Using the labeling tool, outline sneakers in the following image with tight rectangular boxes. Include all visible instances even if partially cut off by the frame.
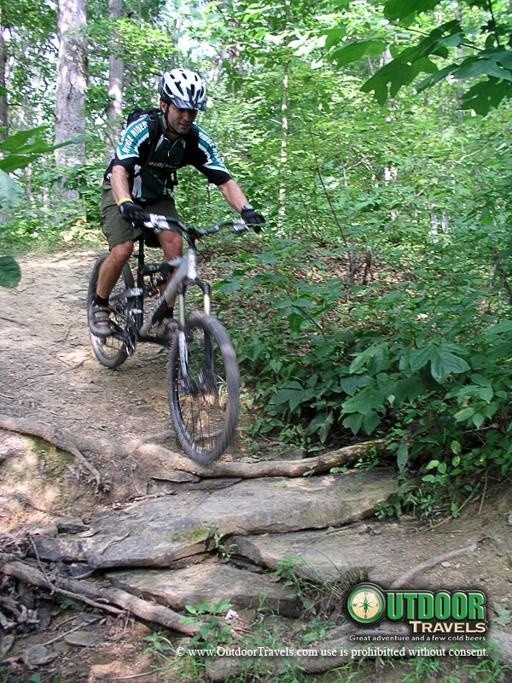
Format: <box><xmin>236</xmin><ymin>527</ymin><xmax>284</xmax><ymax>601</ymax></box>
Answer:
<box><xmin>155</xmin><ymin>317</ymin><xmax>178</xmax><ymax>340</ymax></box>
<box><xmin>88</xmin><ymin>302</ymin><xmax>114</xmax><ymax>339</ymax></box>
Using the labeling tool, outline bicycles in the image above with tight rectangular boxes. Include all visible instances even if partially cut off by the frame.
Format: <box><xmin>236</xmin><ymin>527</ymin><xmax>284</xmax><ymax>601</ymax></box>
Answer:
<box><xmin>87</xmin><ymin>205</ymin><xmax>264</xmax><ymax>466</ymax></box>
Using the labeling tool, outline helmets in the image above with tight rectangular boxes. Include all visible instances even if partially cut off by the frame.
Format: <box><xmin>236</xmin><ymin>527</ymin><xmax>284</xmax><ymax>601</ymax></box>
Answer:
<box><xmin>159</xmin><ymin>69</ymin><xmax>207</xmax><ymax>112</ymax></box>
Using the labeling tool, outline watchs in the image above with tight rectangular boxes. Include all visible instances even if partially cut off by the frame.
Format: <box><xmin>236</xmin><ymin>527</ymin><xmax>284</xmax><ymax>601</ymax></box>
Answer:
<box><xmin>241</xmin><ymin>203</ymin><xmax>255</xmax><ymax>215</ymax></box>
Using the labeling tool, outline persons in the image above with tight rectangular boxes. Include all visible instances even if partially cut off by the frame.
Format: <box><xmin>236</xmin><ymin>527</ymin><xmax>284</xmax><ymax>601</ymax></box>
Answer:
<box><xmin>88</xmin><ymin>68</ymin><xmax>265</xmax><ymax>343</ymax></box>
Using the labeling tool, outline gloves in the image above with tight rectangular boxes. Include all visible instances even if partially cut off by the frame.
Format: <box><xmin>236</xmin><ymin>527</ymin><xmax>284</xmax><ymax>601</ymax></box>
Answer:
<box><xmin>117</xmin><ymin>201</ymin><xmax>145</xmax><ymax>222</ymax></box>
<box><xmin>241</xmin><ymin>205</ymin><xmax>266</xmax><ymax>233</ymax></box>
